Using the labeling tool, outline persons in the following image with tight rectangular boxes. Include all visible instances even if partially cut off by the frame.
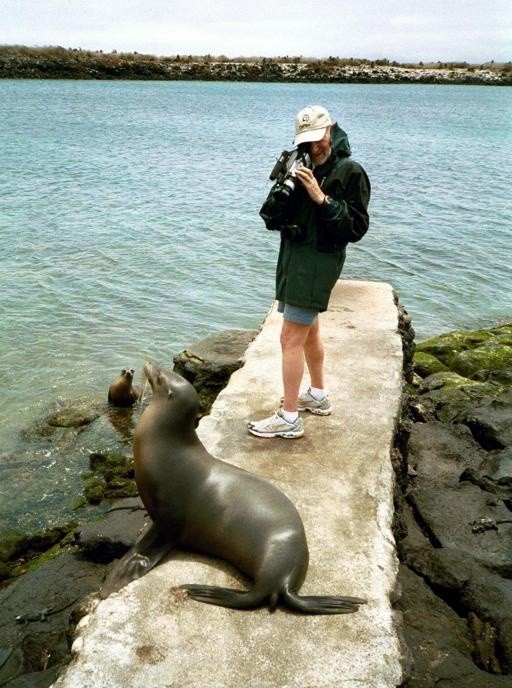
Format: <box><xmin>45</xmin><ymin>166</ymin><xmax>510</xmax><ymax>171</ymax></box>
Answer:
<box><xmin>243</xmin><ymin>104</ymin><xmax>371</xmax><ymax>439</ymax></box>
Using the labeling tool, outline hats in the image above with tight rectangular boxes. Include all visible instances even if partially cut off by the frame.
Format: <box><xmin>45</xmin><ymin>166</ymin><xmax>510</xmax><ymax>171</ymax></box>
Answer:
<box><xmin>293</xmin><ymin>105</ymin><xmax>333</xmax><ymax>147</ymax></box>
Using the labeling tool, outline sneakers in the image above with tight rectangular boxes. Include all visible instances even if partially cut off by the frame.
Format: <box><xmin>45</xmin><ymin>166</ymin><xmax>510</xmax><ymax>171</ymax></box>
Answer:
<box><xmin>280</xmin><ymin>388</ymin><xmax>332</xmax><ymax>416</ymax></box>
<box><xmin>247</xmin><ymin>409</ymin><xmax>305</xmax><ymax>439</ymax></box>
